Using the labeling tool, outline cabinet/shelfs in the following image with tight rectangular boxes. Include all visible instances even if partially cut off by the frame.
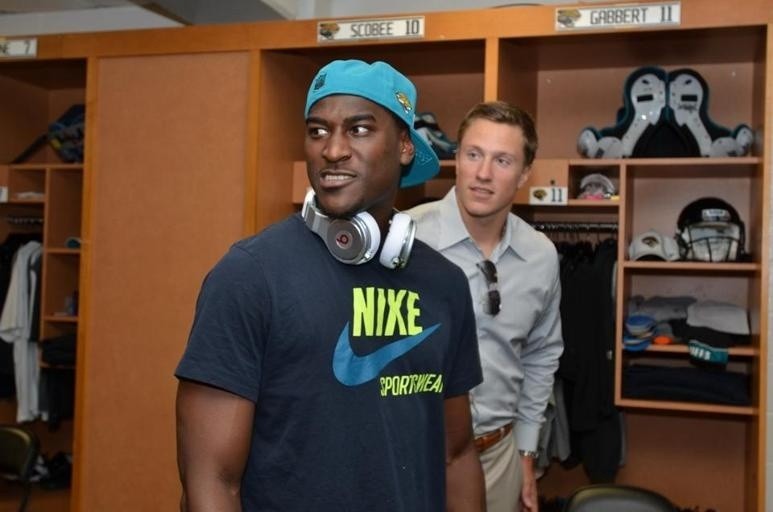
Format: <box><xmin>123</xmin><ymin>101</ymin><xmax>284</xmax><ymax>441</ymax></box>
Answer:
<box><xmin>0</xmin><ymin>0</ymin><xmax>772</xmax><ymax>512</ymax></box>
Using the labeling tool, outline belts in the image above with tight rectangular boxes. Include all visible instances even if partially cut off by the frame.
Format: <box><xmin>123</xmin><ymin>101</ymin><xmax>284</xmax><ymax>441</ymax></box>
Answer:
<box><xmin>474</xmin><ymin>423</ymin><xmax>515</xmax><ymax>452</ymax></box>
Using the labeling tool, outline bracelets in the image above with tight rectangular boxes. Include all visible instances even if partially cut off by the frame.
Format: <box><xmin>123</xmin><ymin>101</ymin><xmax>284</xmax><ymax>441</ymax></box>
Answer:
<box><xmin>519</xmin><ymin>450</ymin><xmax>539</xmax><ymax>459</ymax></box>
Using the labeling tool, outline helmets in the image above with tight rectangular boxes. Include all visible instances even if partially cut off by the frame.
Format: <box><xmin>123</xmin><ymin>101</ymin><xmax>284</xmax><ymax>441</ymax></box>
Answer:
<box><xmin>674</xmin><ymin>197</ymin><xmax>748</xmax><ymax>263</ymax></box>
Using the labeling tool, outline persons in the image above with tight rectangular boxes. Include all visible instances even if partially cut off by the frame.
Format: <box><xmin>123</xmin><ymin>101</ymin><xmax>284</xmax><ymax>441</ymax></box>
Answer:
<box><xmin>172</xmin><ymin>57</ymin><xmax>488</xmax><ymax>512</ymax></box>
<box><xmin>393</xmin><ymin>100</ymin><xmax>564</xmax><ymax>512</ymax></box>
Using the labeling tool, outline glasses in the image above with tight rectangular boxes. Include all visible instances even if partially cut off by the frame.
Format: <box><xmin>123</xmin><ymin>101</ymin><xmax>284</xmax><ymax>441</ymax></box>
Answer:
<box><xmin>476</xmin><ymin>259</ymin><xmax>503</xmax><ymax>317</ymax></box>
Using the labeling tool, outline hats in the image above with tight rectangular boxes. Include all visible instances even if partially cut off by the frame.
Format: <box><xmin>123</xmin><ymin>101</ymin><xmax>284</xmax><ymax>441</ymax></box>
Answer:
<box><xmin>627</xmin><ymin>230</ymin><xmax>674</xmax><ymax>262</ymax></box>
<box><xmin>303</xmin><ymin>58</ymin><xmax>441</xmax><ymax>192</ymax></box>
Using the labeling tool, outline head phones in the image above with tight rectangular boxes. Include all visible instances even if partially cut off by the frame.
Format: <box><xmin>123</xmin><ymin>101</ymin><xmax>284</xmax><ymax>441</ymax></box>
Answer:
<box><xmin>301</xmin><ymin>189</ymin><xmax>418</xmax><ymax>272</ymax></box>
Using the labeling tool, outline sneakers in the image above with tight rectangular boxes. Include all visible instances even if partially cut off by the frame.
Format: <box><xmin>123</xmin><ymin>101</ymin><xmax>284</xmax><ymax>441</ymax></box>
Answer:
<box><xmin>4</xmin><ymin>452</ymin><xmax>73</xmax><ymax>488</ymax></box>
